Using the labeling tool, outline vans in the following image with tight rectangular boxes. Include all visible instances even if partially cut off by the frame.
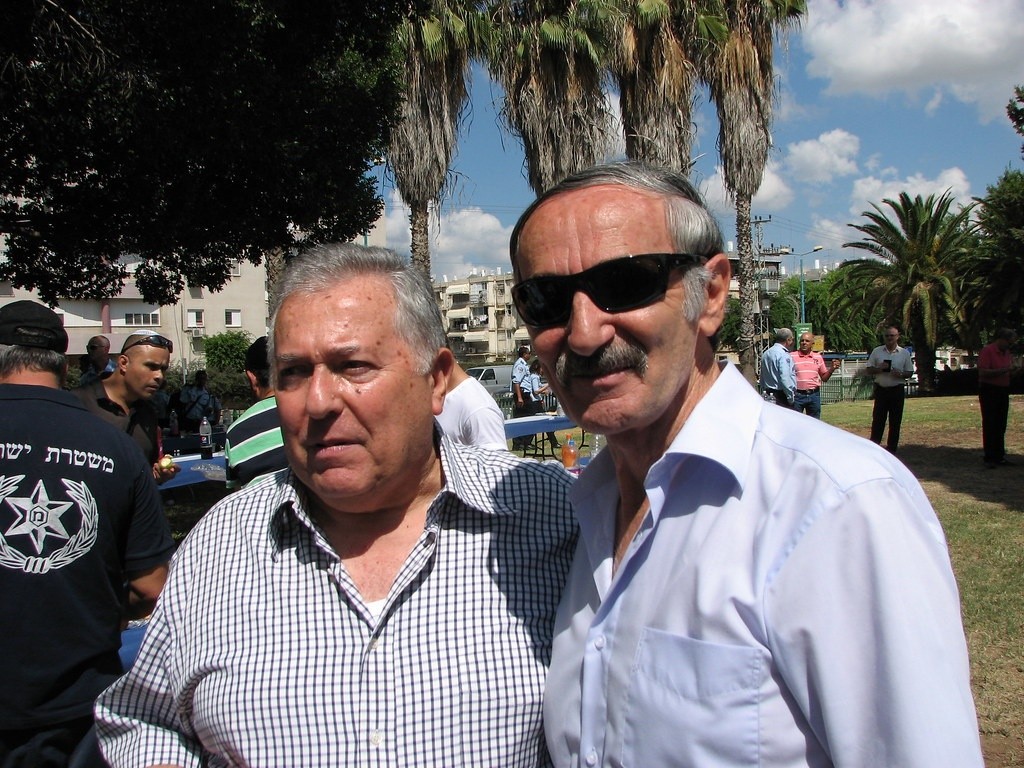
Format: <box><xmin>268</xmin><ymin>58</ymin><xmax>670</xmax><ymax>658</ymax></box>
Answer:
<box><xmin>464</xmin><ymin>363</ymin><xmax>516</xmax><ymax>400</ymax></box>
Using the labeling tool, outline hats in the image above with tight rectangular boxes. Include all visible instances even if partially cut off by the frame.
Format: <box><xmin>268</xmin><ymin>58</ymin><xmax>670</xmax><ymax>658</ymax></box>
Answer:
<box><xmin>0</xmin><ymin>300</ymin><xmax>70</xmax><ymax>351</ymax></box>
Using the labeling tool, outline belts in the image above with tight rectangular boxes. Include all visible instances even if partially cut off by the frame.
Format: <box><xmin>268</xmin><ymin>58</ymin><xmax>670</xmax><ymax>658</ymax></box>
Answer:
<box><xmin>796</xmin><ymin>389</ymin><xmax>819</xmax><ymax>395</ymax></box>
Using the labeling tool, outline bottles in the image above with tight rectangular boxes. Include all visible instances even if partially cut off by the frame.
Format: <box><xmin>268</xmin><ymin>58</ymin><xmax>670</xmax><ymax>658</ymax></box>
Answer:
<box><xmin>169</xmin><ymin>409</ymin><xmax>178</xmax><ymax>438</ymax></box>
<box><xmin>223</xmin><ymin>408</ymin><xmax>237</xmax><ymax>432</ymax></box>
<box><xmin>200</xmin><ymin>416</ymin><xmax>212</xmax><ymax>460</ymax></box>
<box><xmin>561</xmin><ymin>435</ymin><xmax>579</xmax><ymax>467</ymax></box>
<box><xmin>590</xmin><ymin>434</ymin><xmax>607</xmax><ymax>460</ymax></box>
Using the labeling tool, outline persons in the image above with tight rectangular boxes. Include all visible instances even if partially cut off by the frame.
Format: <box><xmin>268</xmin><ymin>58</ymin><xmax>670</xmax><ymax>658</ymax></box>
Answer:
<box><xmin>512</xmin><ymin>347</ymin><xmax>563</xmax><ymax>451</ymax></box>
<box><xmin>866</xmin><ymin>327</ymin><xmax>914</xmax><ymax>456</ymax></box>
<box><xmin>70</xmin><ymin>329</ymin><xmax>183</xmax><ymax>486</ymax></box>
<box><xmin>0</xmin><ymin>300</ymin><xmax>176</xmax><ymax>768</ymax></box>
<box><xmin>510</xmin><ymin>161</ymin><xmax>985</xmax><ymax>768</ymax></box>
<box><xmin>81</xmin><ymin>336</ymin><xmax>116</xmax><ymax>385</ymax></box>
<box><xmin>93</xmin><ymin>243</ymin><xmax>581</xmax><ymax>768</ymax></box>
<box><xmin>225</xmin><ymin>336</ymin><xmax>288</xmax><ymax>490</ymax></box>
<box><xmin>434</xmin><ymin>333</ymin><xmax>508</xmax><ymax>451</ymax></box>
<box><xmin>978</xmin><ymin>329</ymin><xmax>1020</xmax><ymax>468</ymax></box>
<box><xmin>153</xmin><ymin>370</ymin><xmax>222</xmax><ymax>434</ymax></box>
<box><xmin>790</xmin><ymin>333</ymin><xmax>840</xmax><ymax>421</ymax></box>
<box><xmin>760</xmin><ymin>328</ymin><xmax>797</xmax><ymax>410</ymax></box>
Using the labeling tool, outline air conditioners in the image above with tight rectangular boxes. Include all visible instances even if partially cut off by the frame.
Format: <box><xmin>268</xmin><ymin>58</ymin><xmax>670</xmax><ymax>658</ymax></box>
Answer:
<box><xmin>468</xmin><ymin>319</ymin><xmax>476</xmax><ymax>326</ymax></box>
<box><xmin>460</xmin><ymin>324</ymin><xmax>467</xmax><ymax>330</ymax></box>
<box><xmin>469</xmin><ymin>347</ymin><xmax>475</xmax><ymax>353</ymax></box>
<box><xmin>193</xmin><ymin>328</ymin><xmax>206</xmax><ymax>337</ymax></box>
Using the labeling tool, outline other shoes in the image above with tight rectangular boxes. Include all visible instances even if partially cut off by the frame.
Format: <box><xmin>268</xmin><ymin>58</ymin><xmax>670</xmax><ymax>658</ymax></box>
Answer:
<box><xmin>985</xmin><ymin>457</ymin><xmax>995</xmax><ymax>468</ymax></box>
<box><xmin>512</xmin><ymin>444</ymin><xmax>535</xmax><ymax>450</ymax></box>
<box><xmin>997</xmin><ymin>457</ymin><xmax>1007</xmax><ymax>463</ymax></box>
<box><xmin>554</xmin><ymin>444</ymin><xmax>562</xmax><ymax>449</ymax></box>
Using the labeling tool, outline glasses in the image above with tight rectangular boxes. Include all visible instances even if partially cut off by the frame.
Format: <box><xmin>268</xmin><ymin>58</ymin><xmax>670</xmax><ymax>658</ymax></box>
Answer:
<box><xmin>508</xmin><ymin>250</ymin><xmax>708</xmax><ymax>329</ymax></box>
<box><xmin>121</xmin><ymin>333</ymin><xmax>175</xmax><ymax>353</ymax></box>
<box><xmin>885</xmin><ymin>334</ymin><xmax>898</xmax><ymax>337</ymax></box>
<box><xmin>85</xmin><ymin>345</ymin><xmax>106</xmax><ymax>351</ymax></box>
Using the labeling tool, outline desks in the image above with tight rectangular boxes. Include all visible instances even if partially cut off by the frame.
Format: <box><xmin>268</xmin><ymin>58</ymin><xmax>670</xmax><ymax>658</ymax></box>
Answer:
<box><xmin>504</xmin><ymin>411</ymin><xmax>589</xmax><ymax>460</ymax></box>
<box><xmin>158</xmin><ymin>427</ymin><xmax>226</xmax><ymax>502</ymax></box>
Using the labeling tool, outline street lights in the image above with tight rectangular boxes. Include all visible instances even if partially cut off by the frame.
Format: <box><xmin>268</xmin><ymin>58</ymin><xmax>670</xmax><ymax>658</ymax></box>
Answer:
<box><xmin>363</xmin><ymin>156</ymin><xmax>392</xmax><ymax>250</ymax></box>
<box><xmin>781</xmin><ymin>246</ymin><xmax>823</xmax><ymax>325</ymax></box>
